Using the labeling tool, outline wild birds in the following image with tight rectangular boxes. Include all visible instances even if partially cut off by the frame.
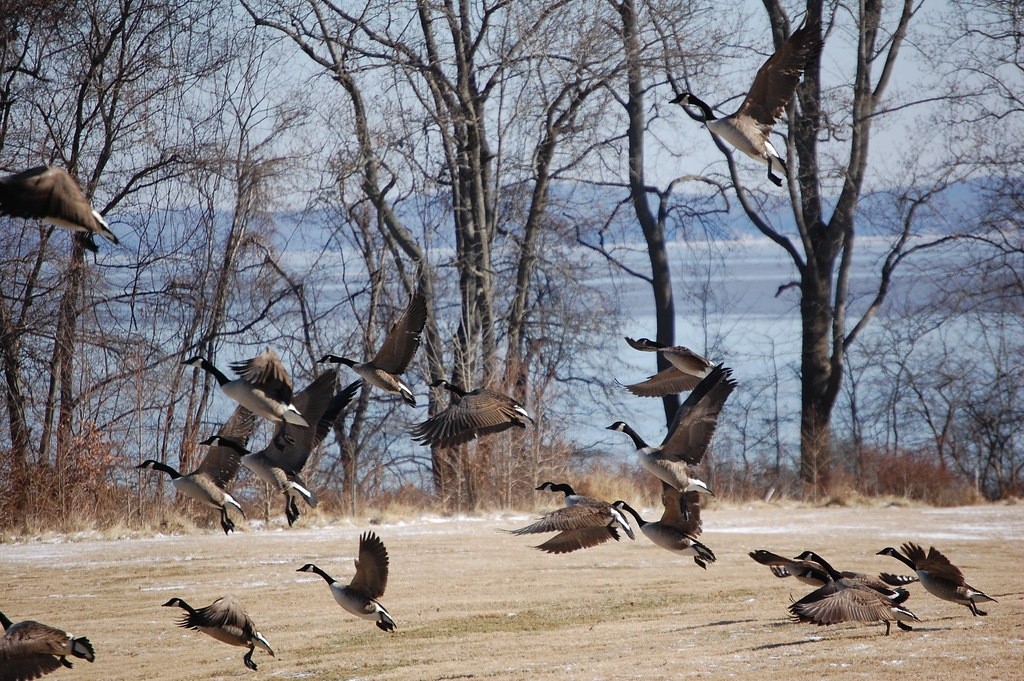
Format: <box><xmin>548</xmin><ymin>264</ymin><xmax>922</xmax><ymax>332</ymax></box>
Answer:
<box><xmin>667</xmin><ymin>9</ymin><xmax>826</xmax><ymax>188</ymax></box>
<box><xmin>296</xmin><ymin>530</ymin><xmax>397</xmax><ymax>632</ymax></box>
<box><xmin>0</xmin><ymin>609</ymin><xmax>96</xmax><ymax>681</ymax></box>
<box><xmin>131</xmin><ymin>276</ymin><xmax>537</xmax><ymax>536</ymax></box>
<box><xmin>495</xmin><ymin>335</ymin><xmax>738</xmax><ymax>570</ymax></box>
<box><xmin>747</xmin><ymin>541</ymin><xmax>998</xmax><ymax>635</ymax></box>
<box><xmin>0</xmin><ymin>166</ymin><xmax>120</xmax><ymax>252</ymax></box>
<box><xmin>160</xmin><ymin>593</ymin><xmax>275</xmax><ymax>673</ymax></box>
